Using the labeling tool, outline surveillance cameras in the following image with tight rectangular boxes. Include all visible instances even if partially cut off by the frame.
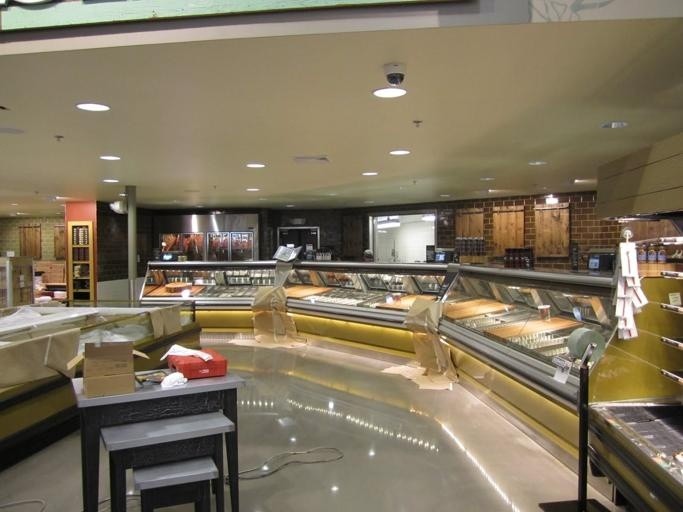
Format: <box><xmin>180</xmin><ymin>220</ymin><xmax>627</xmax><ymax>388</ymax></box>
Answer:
<box><xmin>383</xmin><ymin>62</ymin><xmax>406</xmax><ymax>87</ymax></box>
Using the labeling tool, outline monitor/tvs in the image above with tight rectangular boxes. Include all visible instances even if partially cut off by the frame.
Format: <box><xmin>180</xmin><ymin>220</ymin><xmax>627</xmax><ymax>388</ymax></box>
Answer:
<box><xmin>435</xmin><ymin>247</ymin><xmax>459</xmax><ymax>263</ymax></box>
<box><xmin>161</xmin><ymin>251</ymin><xmax>184</xmax><ymax>261</ymax></box>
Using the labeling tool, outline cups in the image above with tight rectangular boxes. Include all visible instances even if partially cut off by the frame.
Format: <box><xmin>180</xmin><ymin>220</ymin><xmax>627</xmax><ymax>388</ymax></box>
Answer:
<box><xmin>315</xmin><ymin>248</ymin><xmax>332</xmax><ymax>260</ymax></box>
<box><xmin>538</xmin><ymin>303</ymin><xmax>551</xmax><ymax>322</ymax></box>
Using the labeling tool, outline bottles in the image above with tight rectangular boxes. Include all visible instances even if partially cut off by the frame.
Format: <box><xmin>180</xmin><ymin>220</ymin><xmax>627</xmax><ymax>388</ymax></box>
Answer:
<box><xmin>504</xmin><ymin>248</ymin><xmax>534</xmax><ymax>269</ymax></box>
<box><xmin>635</xmin><ymin>243</ymin><xmax>669</xmax><ymax>264</ymax></box>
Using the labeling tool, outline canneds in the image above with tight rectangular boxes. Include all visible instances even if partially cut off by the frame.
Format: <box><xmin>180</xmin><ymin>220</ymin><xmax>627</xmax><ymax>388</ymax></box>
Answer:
<box><xmin>538</xmin><ymin>305</ymin><xmax>551</xmax><ymax>322</ymax></box>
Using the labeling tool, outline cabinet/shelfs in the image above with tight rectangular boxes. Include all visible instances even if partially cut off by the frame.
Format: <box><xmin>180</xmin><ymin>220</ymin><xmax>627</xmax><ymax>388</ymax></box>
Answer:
<box><xmin>0</xmin><ymin>220</ymin><xmax>683</xmax><ymax>512</ymax></box>
